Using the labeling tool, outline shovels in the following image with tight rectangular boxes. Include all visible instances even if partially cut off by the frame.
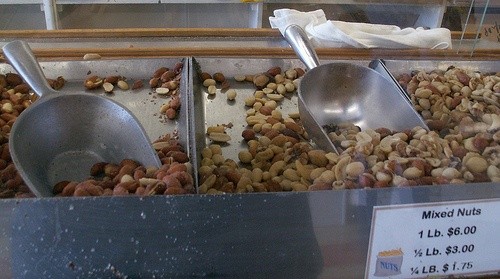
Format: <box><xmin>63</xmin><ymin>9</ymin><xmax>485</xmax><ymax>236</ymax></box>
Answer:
<box><xmin>0</xmin><ymin>37</ymin><xmax>163</xmax><ymax>199</ymax></box>
<box><xmin>283</xmin><ymin>23</ymin><xmax>431</xmax><ymax>159</ymax></box>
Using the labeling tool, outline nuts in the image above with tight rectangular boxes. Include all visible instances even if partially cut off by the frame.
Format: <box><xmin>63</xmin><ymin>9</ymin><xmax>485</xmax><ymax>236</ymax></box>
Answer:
<box><xmin>0</xmin><ymin>53</ymin><xmax>500</xmax><ymax>197</ymax></box>
